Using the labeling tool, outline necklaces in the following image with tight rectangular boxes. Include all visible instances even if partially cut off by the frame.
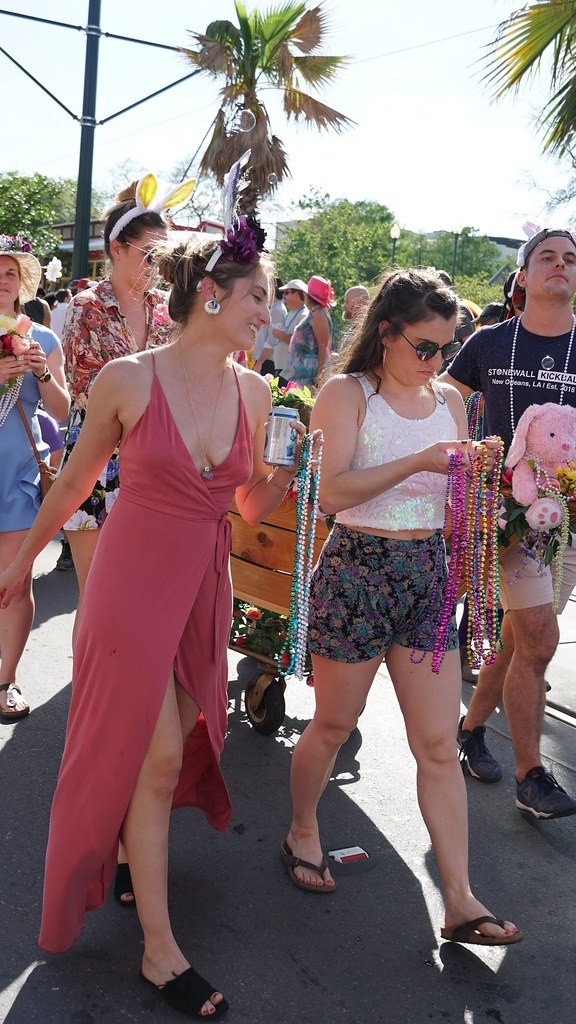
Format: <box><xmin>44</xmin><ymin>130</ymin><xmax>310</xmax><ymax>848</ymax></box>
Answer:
<box><xmin>407</xmin><ymin>436</ymin><xmax>506</xmax><ymax>674</ymax></box>
<box><xmin>465</xmin><ymin>391</ymin><xmax>576</xmax><ymax>502</ymax></box>
<box><xmin>510</xmin><ymin>310</ymin><xmax>576</xmax><ymax>438</ymax></box>
<box><xmin>178</xmin><ymin>335</ymin><xmax>227</xmax><ymax>482</ymax></box>
<box><xmin>274</xmin><ymin>306</ymin><xmax>305</xmax><ymax>346</ymax></box>
<box><xmin>0</xmin><ymin>324</ymin><xmax>25</xmax><ymax>427</ymax></box>
<box><xmin>279</xmin><ymin>430</ymin><xmax>324</xmax><ymax>682</ymax></box>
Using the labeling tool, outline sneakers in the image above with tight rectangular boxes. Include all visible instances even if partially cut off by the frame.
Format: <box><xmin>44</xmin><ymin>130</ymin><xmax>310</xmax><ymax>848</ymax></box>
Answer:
<box><xmin>515</xmin><ymin>766</ymin><xmax>576</xmax><ymax>820</ymax></box>
<box><xmin>56</xmin><ymin>538</ymin><xmax>75</xmax><ymax>571</ymax></box>
<box><xmin>455</xmin><ymin>715</ymin><xmax>503</xmax><ymax>781</ymax></box>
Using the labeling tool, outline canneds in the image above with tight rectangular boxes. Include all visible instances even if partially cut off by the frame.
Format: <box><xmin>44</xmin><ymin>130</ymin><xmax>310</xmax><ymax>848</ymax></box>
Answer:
<box><xmin>263</xmin><ymin>406</ymin><xmax>299</xmax><ymax>467</ymax></box>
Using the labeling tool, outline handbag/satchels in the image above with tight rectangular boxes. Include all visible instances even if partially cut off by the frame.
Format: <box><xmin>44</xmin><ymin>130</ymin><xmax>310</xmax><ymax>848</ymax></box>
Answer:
<box><xmin>39</xmin><ymin>461</ymin><xmax>58</xmax><ymax>504</ymax></box>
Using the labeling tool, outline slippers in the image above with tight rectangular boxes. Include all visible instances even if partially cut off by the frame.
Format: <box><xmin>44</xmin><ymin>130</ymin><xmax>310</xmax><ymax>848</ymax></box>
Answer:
<box><xmin>137</xmin><ymin>965</ymin><xmax>230</xmax><ymax>1021</ymax></box>
<box><xmin>0</xmin><ymin>683</ymin><xmax>29</xmax><ymax>718</ymax></box>
<box><xmin>280</xmin><ymin>839</ymin><xmax>338</xmax><ymax>894</ymax></box>
<box><xmin>440</xmin><ymin>916</ymin><xmax>523</xmax><ymax>945</ymax></box>
<box><xmin>115</xmin><ymin>858</ymin><xmax>137</xmax><ymax>907</ymax></box>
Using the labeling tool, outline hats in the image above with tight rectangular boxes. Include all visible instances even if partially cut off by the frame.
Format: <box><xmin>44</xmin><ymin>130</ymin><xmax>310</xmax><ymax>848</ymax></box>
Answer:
<box><xmin>303</xmin><ymin>276</ymin><xmax>331</xmax><ymax>310</ymax></box>
<box><xmin>0</xmin><ymin>233</ymin><xmax>41</xmax><ymax>307</ymax></box>
<box><xmin>74</xmin><ymin>278</ymin><xmax>90</xmax><ymax>289</ymax></box>
<box><xmin>512</xmin><ymin>229</ymin><xmax>576</xmax><ymax>312</ymax></box>
<box><xmin>277</xmin><ymin>279</ymin><xmax>307</xmax><ymax>292</ymax></box>
<box><xmin>470</xmin><ymin>303</ymin><xmax>509</xmax><ymax>324</ymax></box>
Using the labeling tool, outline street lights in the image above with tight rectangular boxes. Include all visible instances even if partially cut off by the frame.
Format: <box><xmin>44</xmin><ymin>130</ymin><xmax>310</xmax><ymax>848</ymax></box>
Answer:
<box><xmin>452</xmin><ymin>222</ymin><xmax>464</xmax><ymax>283</ymax></box>
<box><xmin>390</xmin><ymin>222</ymin><xmax>401</xmax><ymax>266</ymax></box>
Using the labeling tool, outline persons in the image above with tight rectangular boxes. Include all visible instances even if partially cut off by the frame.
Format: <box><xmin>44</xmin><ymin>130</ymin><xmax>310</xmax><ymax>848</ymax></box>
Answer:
<box><xmin>22</xmin><ymin>278</ymin><xmax>98</xmax><ymax>344</ymax></box>
<box><xmin>62</xmin><ymin>179</ymin><xmax>183</xmax><ymax>644</ymax></box>
<box><xmin>0</xmin><ymin>251</ymin><xmax>71</xmax><ymax>717</ymax></box>
<box><xmin>281</xmin><ymin>269</ymin><xmax>525</xmax><ymax>945</ymax></box>
<box><xmin>437</xmin><ymin>229</ymin><xmax>576</xmax><ymax>820</ymax></box>
<box><xmin>0</xmin><ymin>239</ymin><xmax>323</xmax><ymax>1024</ymax></box>
<box><xmin>435</xmin><ymin>270</ymin><xmax>527</xmax><ymax>373</ymax></box>
<box><xmin>234</xmin><ymin>271</ymin><xmax>370</xmax><ymax>387</ymax></box>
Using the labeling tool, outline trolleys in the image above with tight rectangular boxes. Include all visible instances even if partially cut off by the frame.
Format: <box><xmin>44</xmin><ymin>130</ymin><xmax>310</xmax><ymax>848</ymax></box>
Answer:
<box><xmin>228</xmin><ymin>601</ymin><xmax>316</xmax><ymax>736</ymax></box>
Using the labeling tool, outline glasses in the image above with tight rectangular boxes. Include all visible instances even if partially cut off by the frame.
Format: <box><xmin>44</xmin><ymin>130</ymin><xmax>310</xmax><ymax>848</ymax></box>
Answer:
<box><xmin>120</xmin><ymin>239</ymin><xmax>161</xmax><ymax>267</ymax></box>
<box><xmin>388</xmin><ymin>321</ymin><xmax>461</xmax><ymax>361</ymax></box>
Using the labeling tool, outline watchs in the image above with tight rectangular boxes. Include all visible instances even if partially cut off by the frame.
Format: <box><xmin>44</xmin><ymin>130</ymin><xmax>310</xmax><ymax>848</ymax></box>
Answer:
<box><xmin>33</xmin><ymin>367</ymin><xmax>51</xmax><ymax>383</ymax></box>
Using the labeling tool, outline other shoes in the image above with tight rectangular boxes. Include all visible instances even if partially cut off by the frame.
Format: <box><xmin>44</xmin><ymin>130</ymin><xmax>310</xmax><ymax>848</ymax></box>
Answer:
<box><xmin>544</xmin><ymin>680</ymin><xmax>552</xmax><ymax>693</ymax></box>
<box><xmin>458</xmin><ymin>644</ymin><xmax>481</xmax><ymax>683</ymax></box>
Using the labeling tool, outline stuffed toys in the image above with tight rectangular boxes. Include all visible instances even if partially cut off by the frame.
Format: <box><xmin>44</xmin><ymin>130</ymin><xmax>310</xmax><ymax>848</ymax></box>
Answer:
<box><xmin>503</xmin><ymin>402</ymin><xmax>576</xmax><ymax>531</ymax></box>
